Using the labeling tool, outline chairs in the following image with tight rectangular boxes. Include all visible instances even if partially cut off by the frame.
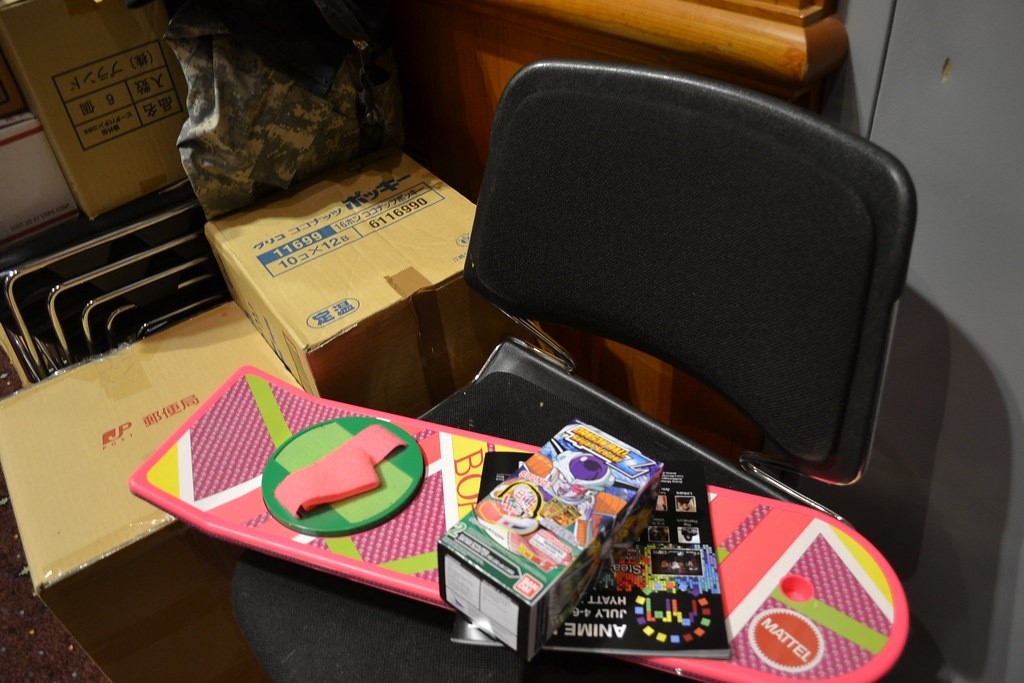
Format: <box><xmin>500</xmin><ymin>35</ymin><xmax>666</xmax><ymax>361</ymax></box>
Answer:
<box><xmin>229</xmin><ymin>58</ymin><xmax>917</xmax><ymax>683</ymax></box>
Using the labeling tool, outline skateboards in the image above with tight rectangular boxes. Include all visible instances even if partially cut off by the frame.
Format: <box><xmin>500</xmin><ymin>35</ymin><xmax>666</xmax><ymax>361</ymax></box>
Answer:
<box><xmin>125</xmin><ymin>362</ymin><xmax>910</xmax><ymax>683</ymax></box>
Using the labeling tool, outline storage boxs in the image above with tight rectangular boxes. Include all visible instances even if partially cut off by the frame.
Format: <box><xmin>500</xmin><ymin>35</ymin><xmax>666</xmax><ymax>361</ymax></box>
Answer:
<box><xmin>0</xmin><ymin>0</ymin><xmax>188</xmax><ymax>255</ymax></box>
<box><xmin>203</xmin><ymin>154</ymin><xmax>534</xmax><ymax>419</ymax></box>
<box><xmin>0</xmin><ymin>301</ymin><xmax>305</xmax><ymax>683</ymax></box>
<box><xmin>438</xmin><ymin>416</ymin><xmax>666</xmax><ymax>661</ymax></box>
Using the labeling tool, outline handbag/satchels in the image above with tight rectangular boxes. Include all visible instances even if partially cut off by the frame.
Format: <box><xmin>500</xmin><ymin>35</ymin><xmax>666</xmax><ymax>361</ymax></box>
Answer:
<box><xmin>162</xmin><ymin>13</ymin><xmax>405</xmax><ymax>221</ymax></box>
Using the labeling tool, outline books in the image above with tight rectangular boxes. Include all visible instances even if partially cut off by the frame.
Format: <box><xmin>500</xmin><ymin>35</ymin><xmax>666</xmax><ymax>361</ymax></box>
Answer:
<box><xmin>449</xmin><ymin>452</ymin><xmax>729</xmax><ymax>660</ymax></box>
